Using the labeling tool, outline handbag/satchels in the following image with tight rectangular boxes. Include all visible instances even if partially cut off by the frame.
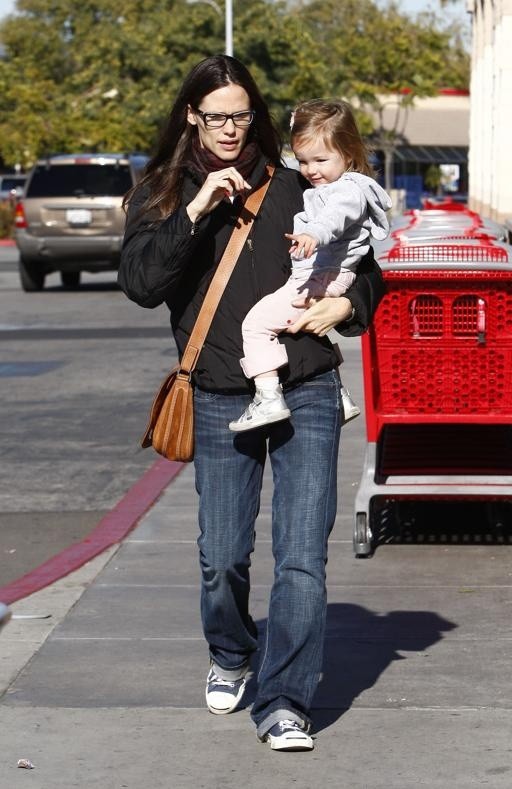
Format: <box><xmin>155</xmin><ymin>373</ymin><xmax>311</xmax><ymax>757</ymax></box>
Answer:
<box><xmin>139</xmin><ymin>366</ymin><xmax>195</xmax><ymax>464</ymax></box>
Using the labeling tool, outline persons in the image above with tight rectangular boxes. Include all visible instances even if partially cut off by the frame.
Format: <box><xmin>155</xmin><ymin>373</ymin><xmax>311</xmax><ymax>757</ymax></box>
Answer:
<box><xmin>118</xmin><ymin>54</ymin><xmax>393</xmax><ymax>750</ymax></box>
<box><xmin>228</xmin><ymin>96</ymin><xmax>393</xmax><ymax>432</ymax></box>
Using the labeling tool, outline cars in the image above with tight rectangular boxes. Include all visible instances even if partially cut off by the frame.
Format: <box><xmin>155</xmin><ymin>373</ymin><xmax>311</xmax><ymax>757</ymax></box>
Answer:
<box><xmin>1</xmin><ymin>175</ymin><xmax>28</xmax><ymax>204</ymax></box>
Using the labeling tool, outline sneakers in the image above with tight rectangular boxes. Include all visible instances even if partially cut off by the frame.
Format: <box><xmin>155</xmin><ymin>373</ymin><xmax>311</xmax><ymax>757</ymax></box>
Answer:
<box><xmin>340</xmin><ymin>387</ymin><xmax>361</xmax><ymax>425</ymax></box>
<box><xmin>228</xmin><ymin>384</ymin><xmax>291</xmax><ymax>433</ymax></box>
<box><xmin>263</xmin><ymin>720</ymin><xmax>315</xmax><ymax>751</ymax></box>
<box><xmin>205</xmin><ymin>663</ymin><xmax>249</xmax><ymax>716</ymax></box>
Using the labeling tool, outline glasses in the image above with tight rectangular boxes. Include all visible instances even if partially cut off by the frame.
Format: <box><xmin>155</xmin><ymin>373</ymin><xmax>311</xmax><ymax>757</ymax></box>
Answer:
<box><xmin>193</xmin><ymin>105</ymin><xmax>256</xmax><ymax>127</ymax></box>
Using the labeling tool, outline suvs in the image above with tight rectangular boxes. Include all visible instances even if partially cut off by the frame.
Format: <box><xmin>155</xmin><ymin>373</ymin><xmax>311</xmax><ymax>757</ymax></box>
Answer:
<box><xmin>14</xmin><ymin>150</ymin><xmax>153</xmax><ymax>291</ymax></box>
